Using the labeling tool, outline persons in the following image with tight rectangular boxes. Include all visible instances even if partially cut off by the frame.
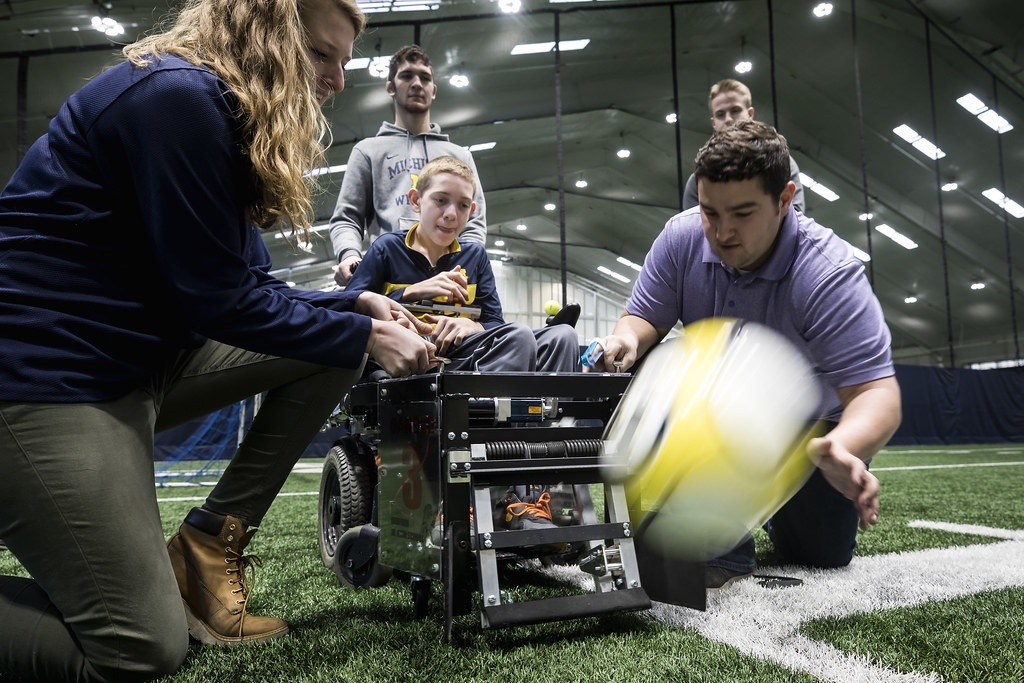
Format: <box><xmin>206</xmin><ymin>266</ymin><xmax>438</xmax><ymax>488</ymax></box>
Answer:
<box><xmin>338</xmin><ymin>154</ymin><xmax>579</xmax><ymax>551</ymax></box>
<box><xmin>681</xmin><ymin>79</ymin><xmax>805</xmax><ymax>215</ymax></box>
<box><xmin>0</xmin><ymin>0</ymin><xmax>437</xmax><ymax>683</ymax></box>
<box><xmin>583</xmin><ymin>120</ymin><xmax>902</xmax><ymax>588</ymax></box>
<box><xmin>326</xmin><ymin>44</ymin><xmax>486</xmax><ymax>285</ymax></box>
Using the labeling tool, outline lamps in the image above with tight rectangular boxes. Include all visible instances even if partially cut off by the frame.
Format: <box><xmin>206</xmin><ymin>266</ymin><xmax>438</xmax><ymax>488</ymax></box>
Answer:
<box><xmin>733</xmin><ymin>37</ymin><xmax>753</xmax><ymax>74</ymax></box>
<box><xmin>543</xmin><ymin>190</ymin><xmax>556</xmax><ymax>210</ymax></box>
<box><xmin>666</xmin><ymin>99</ymin><xmax>678</xmax><ymax>123</ymax></box>
<box><xmin>494</xmin><ymin>225</ymin><xmax>505</xmax><ymax>247</ymax></box>
<box><xmin>575</xmin><ymin>173</ymin><xmax>588</xmax><ymax>188</ymax></box>
<box><xmin>968</xmin><ymin>276</ymin><xmax>986</xmax><ymax>290</ymax></box>
<box><xmin>903</xmin><ymin>293</ymin><xmax>918</xmax><ymax>304</ymax></box>
<box><xmin>940</xmin><ymin>176</ymin><xmax>958</xmax><ymax>192</ymax></box>
<box><xmin>616</xmin><ymin>131</ymin><xmax>631</xmax><ymax>159</ymax></box>
<box><xmin>368</xmin><ymin>42</ymin><xmax>391</xmax><ymax>78</ymax></box>
<box><xmin>858</xmin><ymin>208</ymin><xmax>873</xmax><ymax>221</ymax></box>
<box><xmin>448</xmin><ymin>62</ymin><xmax>469</xmax><ymax>88</ymax></box>
<box><xmin>516</xmin><ymin>215</ymin><xmax>528</xmax><ymax>230</ymax></box>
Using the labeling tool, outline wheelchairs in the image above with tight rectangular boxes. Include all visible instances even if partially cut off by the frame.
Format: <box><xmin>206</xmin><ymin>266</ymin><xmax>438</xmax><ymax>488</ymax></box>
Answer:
<box><xmin>318</xmin><ymin>261</ymin><xmax>652</xmax><ymax>644</ymax></box>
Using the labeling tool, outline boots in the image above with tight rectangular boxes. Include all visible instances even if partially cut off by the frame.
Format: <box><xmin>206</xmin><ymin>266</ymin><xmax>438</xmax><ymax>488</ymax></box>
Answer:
<box><xmin>166</xmin><ymin>507</ymin><xmax>289</xmax><ymax>643</ymax></box>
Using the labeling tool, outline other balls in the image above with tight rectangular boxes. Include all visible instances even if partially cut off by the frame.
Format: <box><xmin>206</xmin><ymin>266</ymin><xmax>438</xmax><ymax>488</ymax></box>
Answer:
<box><xmin>581</xmin><ymin>311</ymin><xmax>844</xmax><ymax>576</ymax></box>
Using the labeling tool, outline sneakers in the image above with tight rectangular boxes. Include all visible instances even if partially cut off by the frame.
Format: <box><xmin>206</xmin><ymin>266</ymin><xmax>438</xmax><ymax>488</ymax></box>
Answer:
<box><xmin>491</xmin><ymin>491</ymin><xmax>567</xmax><ymax>553</ymax></box>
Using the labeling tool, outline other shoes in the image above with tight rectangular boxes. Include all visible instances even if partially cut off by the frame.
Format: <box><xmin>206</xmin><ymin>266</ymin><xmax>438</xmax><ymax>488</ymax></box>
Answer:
<box><xmin>702</xmin><ymin>563</ymin><xmax>758</xmax><ymax>591</ymax></box>
